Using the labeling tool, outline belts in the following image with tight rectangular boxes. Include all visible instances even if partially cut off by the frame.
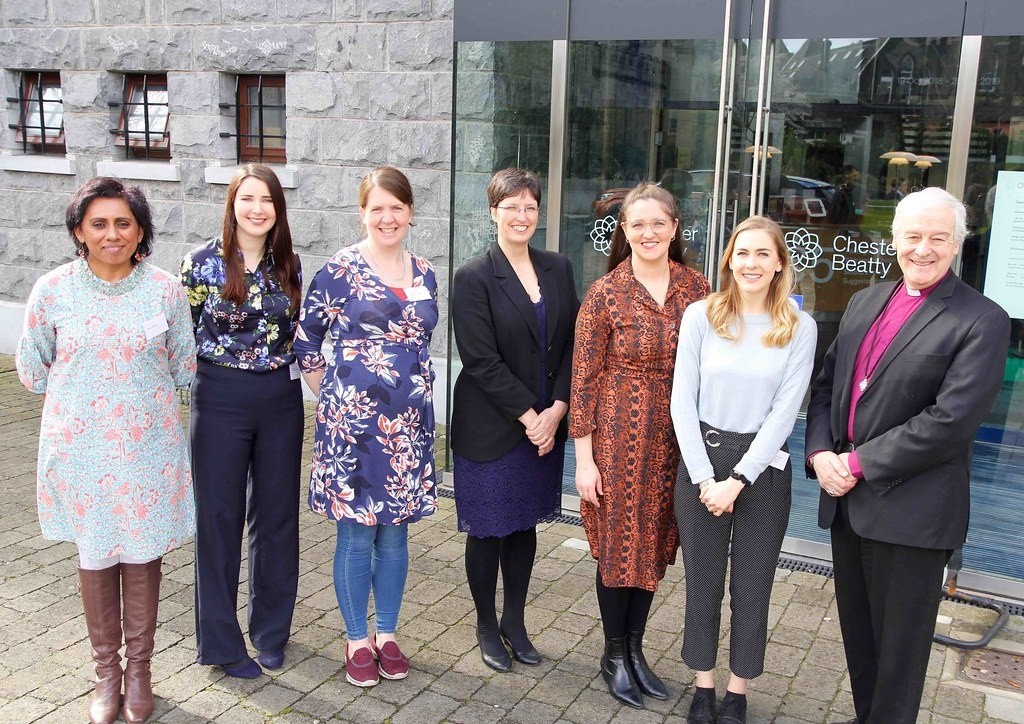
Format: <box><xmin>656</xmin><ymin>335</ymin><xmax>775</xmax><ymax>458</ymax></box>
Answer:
<box><xmin>843</xmin><ymin>443</ymin><xmax>855</xmax><ymax>452</ymax></box>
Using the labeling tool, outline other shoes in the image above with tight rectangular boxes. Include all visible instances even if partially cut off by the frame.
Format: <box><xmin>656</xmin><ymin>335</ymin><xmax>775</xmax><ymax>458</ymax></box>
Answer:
<box><xmin>370</xmin><ymin>632</ymin><xmax>409</xmax><ymax>679</ymax></box>
<box><xmin>257</xmin><ymin>645</ymin><xmax>284</xmax><ymax>669</ymax></box>
<box><xmin>221</xmin><ymin>654</ymin><xmax>262</xmax><ymax>678</ymax></box>
<box><xmin>343</xmin><ymin>642</ymin><xmax>380</xmax><ymax>687</ymax></box>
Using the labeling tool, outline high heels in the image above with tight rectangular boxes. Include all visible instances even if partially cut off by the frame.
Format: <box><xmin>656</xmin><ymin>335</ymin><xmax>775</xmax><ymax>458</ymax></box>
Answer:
<box><xmin>475</xmin><ymin>624</ymin><xmax>511</xmax><ymax>672</ymax></box>
<box><xmin>499</xmin><ymin>619</ymin><xmax>541</xmax><ymax>664</ymax></box>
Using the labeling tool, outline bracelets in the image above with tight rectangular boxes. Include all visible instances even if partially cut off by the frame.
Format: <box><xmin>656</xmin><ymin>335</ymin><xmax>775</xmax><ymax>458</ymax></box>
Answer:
<box><xmin>730</xmin><ymin>470</ymin><xmax>751</xmax><ymax>487</ymax></box>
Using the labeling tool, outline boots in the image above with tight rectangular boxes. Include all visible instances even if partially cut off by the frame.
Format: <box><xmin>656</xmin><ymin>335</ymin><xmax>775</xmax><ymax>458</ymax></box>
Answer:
<box><xmin>76</xmin><ymin>561</ymin><xmax>121</xmax><ymax>724</ymax></box>
<box><xmin>600</xmin><ymin>636</ymin><xmax>644</xmax><ymax>709</ymax></box>
<box><xmin>120</xmin><ymin>557</ymin><xmax>163</xmax><ymax>724</ymax></box>
<box><xmin>628</xmin><ymin>629</ymin><xmax>670</xmax><ymax>700</ymax></box>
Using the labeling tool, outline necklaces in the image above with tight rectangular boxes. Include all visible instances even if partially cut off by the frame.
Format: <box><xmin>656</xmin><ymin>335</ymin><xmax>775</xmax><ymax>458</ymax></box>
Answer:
<box><xmin>364</xmin><ymin>242</ymin><xmax>406</xmax><ymax>281</ymax></box>
<box><xmin>859</xmin><ymin>282</ymin><xmax>929</xmax><ymax>392</ymax></box>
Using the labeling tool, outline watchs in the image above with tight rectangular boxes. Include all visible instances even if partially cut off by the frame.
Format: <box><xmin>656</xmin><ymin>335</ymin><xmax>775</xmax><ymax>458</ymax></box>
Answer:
<box><xmin>810</xmin><ymin>456</ymin><xmax>814</xmax><ymax>466</ymax></box>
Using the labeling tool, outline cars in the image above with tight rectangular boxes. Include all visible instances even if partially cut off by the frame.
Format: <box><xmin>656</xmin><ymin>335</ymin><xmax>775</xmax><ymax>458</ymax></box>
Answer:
<box><xmin>590</xmin><ymin>168</ymin><xmax>838</xmax><ymax>241</ymax></box>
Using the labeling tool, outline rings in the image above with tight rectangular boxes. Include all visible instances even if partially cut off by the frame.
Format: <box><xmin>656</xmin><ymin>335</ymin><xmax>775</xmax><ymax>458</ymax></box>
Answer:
<box><xmin>580</xmin><ymin>493</ymin><xmax>583</xmax><ymax>496</ymax></box>
<box><xmin>544</xmin><ymin>437</ymin><xmax>547</xmax><ymax>442</ymax></box>
<box><xmin>828</xmin><ymin>491</ymin><xmax>834</xmax><ymax>495</ymax></box>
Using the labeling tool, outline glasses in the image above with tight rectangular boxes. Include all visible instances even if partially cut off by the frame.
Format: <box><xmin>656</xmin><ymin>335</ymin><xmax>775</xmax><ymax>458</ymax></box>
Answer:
<box><xmin>625</xmin><ymin>217</ymin><xmax>676</xmax><ymax>236</ymax></box>
<box><xmin>496</xmin><ymin>204</ymin><xmax>540</xmax><ymax>218</ymax></box>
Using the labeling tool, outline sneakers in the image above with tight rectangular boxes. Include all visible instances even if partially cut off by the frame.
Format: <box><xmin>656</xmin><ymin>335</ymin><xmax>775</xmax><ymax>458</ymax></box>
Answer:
<box><xmin>687</xmin><ymin>689</ymin><xmax>717</xmax><ymax>724</ymax></box>
<box><xmin>717</xmin><ymin>693</ymin><xmax>748</xmax><ymax>724</ymax></box>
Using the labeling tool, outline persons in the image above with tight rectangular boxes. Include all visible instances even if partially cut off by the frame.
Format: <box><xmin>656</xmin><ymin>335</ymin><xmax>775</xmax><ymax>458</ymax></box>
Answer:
<box><xmin>568</xmin><ymin>185</ymin><xmax>712</xmax><ymax>710</ymax></box>
<box><xmin>826</xmin><ymin>164</ymin><xmax>861</xmax><ymax>223</ymax></box>
<box><xmin>16</xmin><ymin>177</ymin><xmax>198</xmax><ymax>724</ymax></box>
<box><xmin>886</xmin><ymin>177</ymin><xmax>908</xmax><ymax>199</ymax></box>
<box><xmin>177</xmin><ymin>164</ymin><xmax>303</xmax><ymax>678</ymax></box>
<box><xmin>291</xmin><ymin>167</ymin><xmax>440</xmax><ymax>687</ymax></box>
<box><xmin>669</xmin><ymin>215</ymin><xmax>817</xmax><ymax>724</ymax></box>
<box><xmin>451</xmin><ymin>167</ymin><xmax>580</xmax><ymax>672</ymax></box>
<box><xmin>806</xmin><ymin>187</ymin><xmax>1012</xmax><ymax>724</ymax></box>
